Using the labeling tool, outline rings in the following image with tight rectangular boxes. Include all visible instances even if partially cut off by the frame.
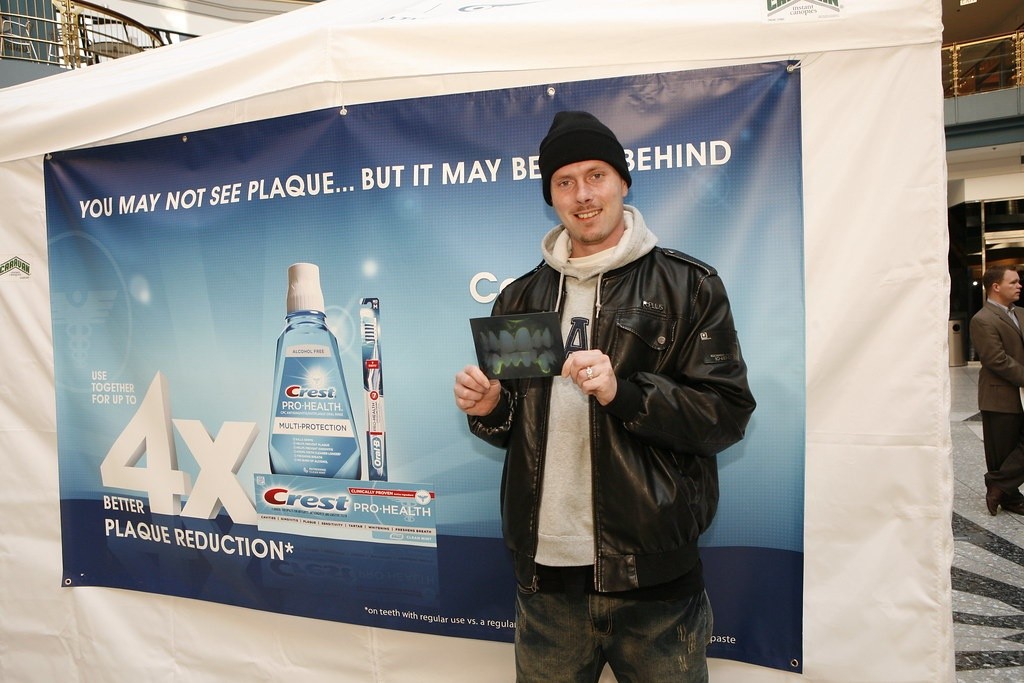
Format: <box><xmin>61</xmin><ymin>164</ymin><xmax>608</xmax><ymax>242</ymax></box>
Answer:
<box><xmin>585</xmin><ymin>367</ymin><xmax>593</xmax><ymax>380</ymax></box>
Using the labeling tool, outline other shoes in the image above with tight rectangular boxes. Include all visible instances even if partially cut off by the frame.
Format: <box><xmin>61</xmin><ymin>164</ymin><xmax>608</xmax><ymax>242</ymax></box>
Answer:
<box><xmin>1000</xmin><ymin>498</ymin><xmax>1024</xmax><ymax>515</ymax></box>
<box><xmin>982</xmin><ymin>473</ymin><xmax>1006</xmax><ymax>517</ymax></box>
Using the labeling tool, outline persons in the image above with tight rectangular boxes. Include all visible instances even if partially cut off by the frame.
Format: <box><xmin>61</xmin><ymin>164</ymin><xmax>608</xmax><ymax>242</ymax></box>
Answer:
<box><xmin>968</xmin><ymin>264</ymin><xmax>1024</xmax><ymax>517</ymax></box>
<box><xmin>453</xmin><ymin>111</ymin><xmax>756</xmax><ymax>683</ymax></box>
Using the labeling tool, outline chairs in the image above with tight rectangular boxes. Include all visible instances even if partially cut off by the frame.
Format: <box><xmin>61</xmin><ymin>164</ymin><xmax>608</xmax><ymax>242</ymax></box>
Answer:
<box><xmin>0</xmin><ymin>19</ymin><xmax>39</xmax><ymax>63</ymax></box>
<box><xmin>48</xmin><ymin>24</ymin><xmax>96</xmax><ymax>69</ymax></box>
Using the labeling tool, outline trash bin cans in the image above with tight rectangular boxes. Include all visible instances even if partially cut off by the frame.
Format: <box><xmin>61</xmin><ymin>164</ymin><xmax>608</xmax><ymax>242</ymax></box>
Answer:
<box><xmin>948</xmin><ymin>319</ymin><xmax>969</xmax><ymax>367</ymax></box>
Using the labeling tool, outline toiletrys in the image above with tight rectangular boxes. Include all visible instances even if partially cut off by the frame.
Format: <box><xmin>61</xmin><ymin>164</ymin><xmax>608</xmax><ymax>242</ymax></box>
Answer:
<box><xmin>268</xmin><ymin>261</ymin><xmax>362</xmax><ymax>480</ymax></box>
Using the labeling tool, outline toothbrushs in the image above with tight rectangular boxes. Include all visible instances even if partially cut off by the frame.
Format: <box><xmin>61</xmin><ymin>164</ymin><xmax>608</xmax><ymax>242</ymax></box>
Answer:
<box><xmin>359</xmin><ymin>307</ymin><xmax>388</xmax><ymax>480</ymax></box>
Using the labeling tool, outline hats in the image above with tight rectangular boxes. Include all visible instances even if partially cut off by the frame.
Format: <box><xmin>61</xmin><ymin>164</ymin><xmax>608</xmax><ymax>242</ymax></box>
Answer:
<box><xmin>538</xmin><ymin>111</ymin><xmax>632</xmax><ymax>206</ymax></box>
